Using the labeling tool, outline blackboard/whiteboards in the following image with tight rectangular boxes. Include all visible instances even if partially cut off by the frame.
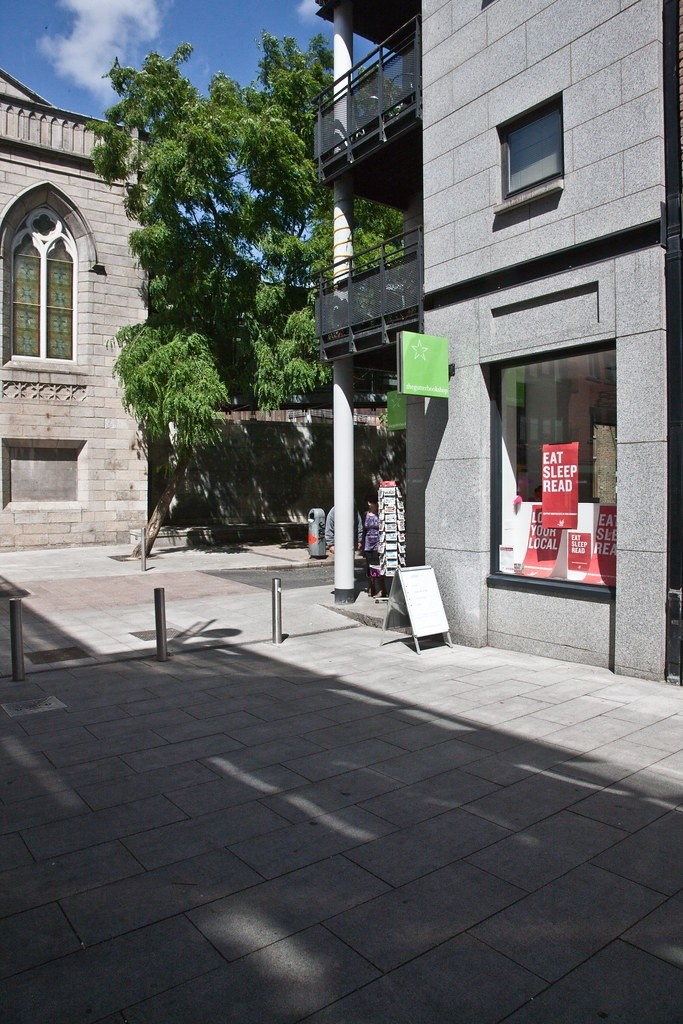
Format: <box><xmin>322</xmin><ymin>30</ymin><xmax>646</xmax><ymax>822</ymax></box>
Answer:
<box><xmin>398</xmin><ymin>565</ymin><xmax>450</xmax><ymax>637</ymax></box>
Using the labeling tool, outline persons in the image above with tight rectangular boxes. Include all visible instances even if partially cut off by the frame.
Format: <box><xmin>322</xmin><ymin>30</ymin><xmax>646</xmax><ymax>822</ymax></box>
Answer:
<box><xmin>325</xmin><ymin>503</ymin><xmax>363</xmax><ymax>594</ymax></box>
<box><xmin>364</xmin><ymin>494</ymin><xmax>386</xmax><ymax>601</ymax></box>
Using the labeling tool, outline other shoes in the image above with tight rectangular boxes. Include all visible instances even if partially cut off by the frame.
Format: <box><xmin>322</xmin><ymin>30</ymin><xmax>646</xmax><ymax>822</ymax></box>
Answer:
<box><xmin>372</xmin><ymin>590</ymin><xmax>382</xmax><ymax>598</ymax></box>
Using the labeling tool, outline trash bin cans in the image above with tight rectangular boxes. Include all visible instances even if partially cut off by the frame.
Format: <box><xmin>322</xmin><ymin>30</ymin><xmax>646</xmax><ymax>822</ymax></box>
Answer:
<box><xmin>308</xmin><ymin>509</ymin><xmax>327</xmax><ymax>558</ymax></box>
